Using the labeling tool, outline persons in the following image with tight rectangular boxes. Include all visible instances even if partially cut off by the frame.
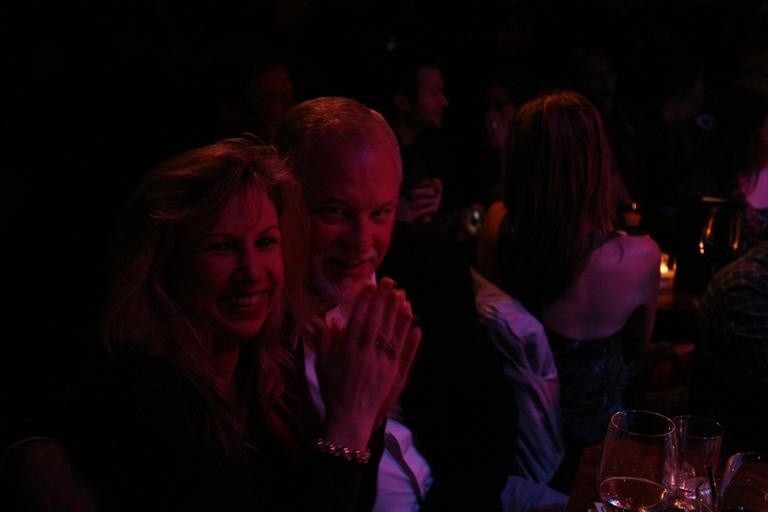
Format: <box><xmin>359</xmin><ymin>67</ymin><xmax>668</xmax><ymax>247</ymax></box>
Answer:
<box><xmin>1</xmin><ymin>0</ymin><xmax>768</xmax><ymax>512</ymax></box>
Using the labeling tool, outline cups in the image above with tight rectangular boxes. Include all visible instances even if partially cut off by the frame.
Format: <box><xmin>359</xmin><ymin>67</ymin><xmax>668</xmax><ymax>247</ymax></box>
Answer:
<box><xmin>590</xmin><ymin>408</ymin><xmax>768</xmax><ymax>512</ymax></box>
